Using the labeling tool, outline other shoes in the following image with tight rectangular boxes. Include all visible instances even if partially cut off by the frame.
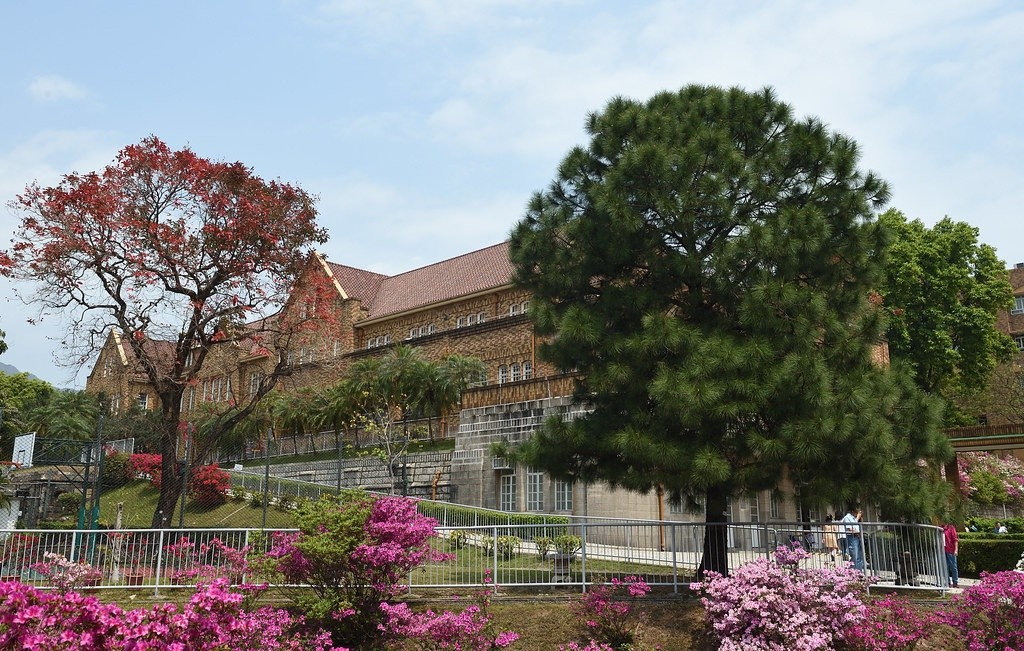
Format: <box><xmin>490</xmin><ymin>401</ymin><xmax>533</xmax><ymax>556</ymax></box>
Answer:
<box><xmin>953</xmin><ymin>584</ymin><xmax>959</xmax><ymax>588</ymax></box>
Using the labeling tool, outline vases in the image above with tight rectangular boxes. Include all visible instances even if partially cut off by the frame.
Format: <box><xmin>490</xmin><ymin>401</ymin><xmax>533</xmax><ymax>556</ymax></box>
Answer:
<box><xmin>228</xmin><ymin>577</ymin><xmax>242</xmax><ymax>585</ymax></box>
<box><xmin>83</xmin><ymin>578</ymin><xmax>101</xmax><ymax>593</ymax></box>
<box><xmin>2</xmin><ymin>575</ymin><xmax>21</xmax><ymax>582</ymax></box>
<box><xmin>169</xmin><ymin>577</ymin><xmax>185</xmax><ymax>592</ymax></box>
<box><xmin>126</xmin><ymin>574</ymin><xmax>143</xmax><ymax>591</ymax></box>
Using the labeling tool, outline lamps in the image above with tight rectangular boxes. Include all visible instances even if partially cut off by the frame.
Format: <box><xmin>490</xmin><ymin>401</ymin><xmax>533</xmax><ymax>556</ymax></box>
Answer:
<box><xmin>440</xmin><ymin>311</ymin><xmax>447</xmax><ymax>320</ymax></box>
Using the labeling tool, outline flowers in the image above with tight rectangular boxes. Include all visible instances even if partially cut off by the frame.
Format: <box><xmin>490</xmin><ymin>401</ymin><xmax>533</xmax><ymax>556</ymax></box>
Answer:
<box><xmin>0</xmin><ymin>533</ymin><xmax>253</xmax><ymax>576</ymax></box>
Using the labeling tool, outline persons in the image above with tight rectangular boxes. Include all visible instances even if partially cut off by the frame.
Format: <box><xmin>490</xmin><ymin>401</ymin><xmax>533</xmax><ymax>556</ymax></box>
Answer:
<box><xmin>823</xmin><ymin>501</ymin><xmax>1008</xmax><ymax>588</ymax></box>
